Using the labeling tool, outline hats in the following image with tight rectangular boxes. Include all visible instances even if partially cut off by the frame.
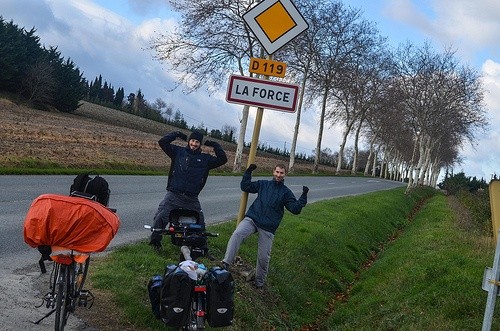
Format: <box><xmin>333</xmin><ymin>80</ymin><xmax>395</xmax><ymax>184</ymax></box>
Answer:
<box><xmin>189</xmin><ymin>132</ymin><xmax>203</xmax><ymax>142</ymax></box>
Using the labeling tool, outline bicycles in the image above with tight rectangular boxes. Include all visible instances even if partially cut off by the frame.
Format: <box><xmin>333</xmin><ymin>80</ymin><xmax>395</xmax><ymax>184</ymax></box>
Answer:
<box><xmin>144</xmin><ymin>206</ymin><xmax>221</xmax><ymax>331</ymax></box>
<box><xmin>28</xmin><ymin>188</ymin><xmax>118</xmax><ymax>331</ymax></box>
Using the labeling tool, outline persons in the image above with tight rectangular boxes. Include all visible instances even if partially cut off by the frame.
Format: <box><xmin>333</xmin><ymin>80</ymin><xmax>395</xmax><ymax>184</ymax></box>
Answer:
<box><xmin>215</xmin><ymin>164</ymin><xmax>309</xmax><ymax>293</ymax></box>
<box><xmin>148</xmin><ymin>130</ymin><xmax>228</xmax><ymax>261</ymax></box>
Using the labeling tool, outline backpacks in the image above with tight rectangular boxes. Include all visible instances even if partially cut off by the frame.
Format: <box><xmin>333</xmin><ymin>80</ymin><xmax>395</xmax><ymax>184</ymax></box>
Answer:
<box><xmin>69</xmin><ymin>171</ymin><xmax>111</xmax><ymax>207</ymax></box>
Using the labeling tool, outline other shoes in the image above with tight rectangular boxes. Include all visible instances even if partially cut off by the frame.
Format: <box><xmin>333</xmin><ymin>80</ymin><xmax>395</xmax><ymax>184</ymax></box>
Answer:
<box><xmin>255</xmin><ymin>280</ymin><xmax>264</xmax><ymax>287</ymax></box>
<box><xmin>215</xmin><ymin>260</ymin><xmax>229</xmax><ymax>270</ymax></box>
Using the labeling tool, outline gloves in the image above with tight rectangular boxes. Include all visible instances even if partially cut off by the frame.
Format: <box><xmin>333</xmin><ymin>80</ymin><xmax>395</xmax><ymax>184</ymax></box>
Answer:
<box><xmin>174</xmin><ymin>130</ymin><xmax>189</xmax><ymax>142</ymax></box>
<box><xmin>247</xmin><ymin>164</ymin><xmax>257</xmax><ymax>171</ymax></box>
<box><xmin>303</xmin><ymin>186</ymin><xmax>309</xmax><ymax>195</ymax></box>
<box><xmin>203</xmin><ymin>140</ymin><xmax>215</xmax><ymax>147</ymax></box>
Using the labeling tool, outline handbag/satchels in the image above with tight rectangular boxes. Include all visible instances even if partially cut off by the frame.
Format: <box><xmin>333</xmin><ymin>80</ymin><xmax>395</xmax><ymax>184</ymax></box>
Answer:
<box><xmin>161</xmin><ymin>265</ymin><xmax>195</xmax><ymax>327</ymax></box>
<box><xmin>205</xmin><ymin>267</ymin><xmax>234</xmax><ymax>326</ymax></box>
<box><xmin>149</xmin><ymin>276</ymin><xmax>164</xmax><ymax>318</ymax></box>
